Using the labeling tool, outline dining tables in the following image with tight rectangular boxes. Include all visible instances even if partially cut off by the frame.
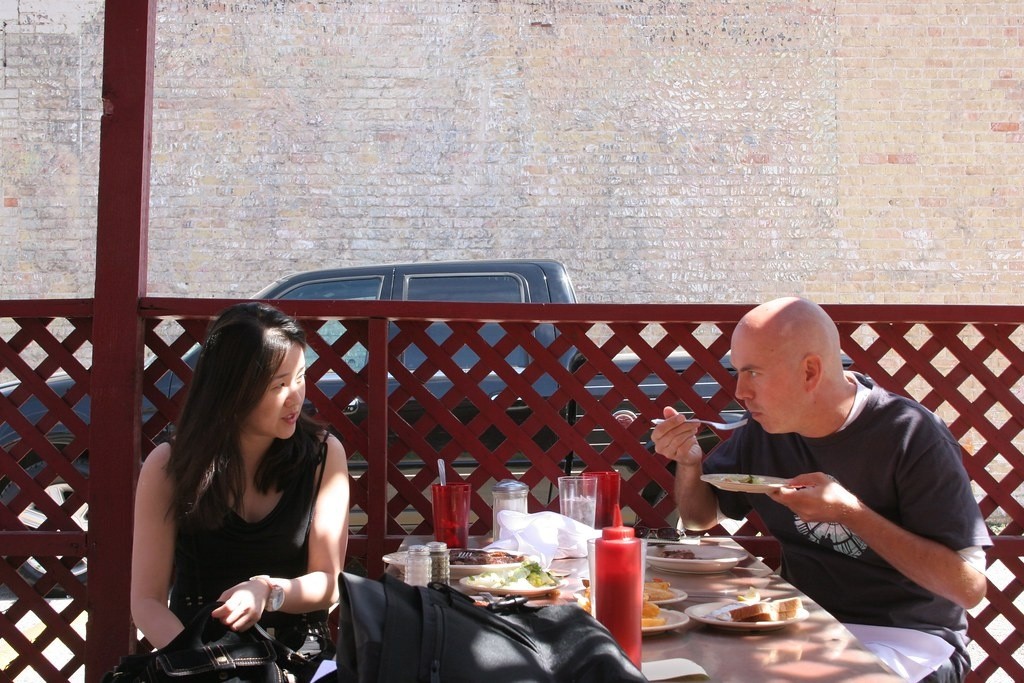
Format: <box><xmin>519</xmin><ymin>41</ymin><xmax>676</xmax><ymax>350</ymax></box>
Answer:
<box><xmin>386</xmin><ymin>535</ymin><xmax>909</xmax><ymax>683</ymax></box>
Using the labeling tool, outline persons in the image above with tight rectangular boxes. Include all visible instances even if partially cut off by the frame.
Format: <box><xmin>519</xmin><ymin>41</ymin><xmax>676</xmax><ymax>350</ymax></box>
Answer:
<box><xmin>651</xmin><ymin>298</ymin><xmax>994</xmax><ymax>683</ymax></box>
<box><xmin>131</xmin><ymin>301</ymin><xmax>349</xmax><ymax>683</ymax></box>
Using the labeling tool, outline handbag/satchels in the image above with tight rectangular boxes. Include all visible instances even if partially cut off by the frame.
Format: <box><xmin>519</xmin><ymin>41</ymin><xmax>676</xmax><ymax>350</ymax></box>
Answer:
<box><xmin>413</xmin><ymin>581</ymin><xmax>649</xmax><ymax>683</ymax></box>
<box><xmin>101</xmin><ymin>601</ymin><xmax>287</xmax><ymax>683</ymax></box>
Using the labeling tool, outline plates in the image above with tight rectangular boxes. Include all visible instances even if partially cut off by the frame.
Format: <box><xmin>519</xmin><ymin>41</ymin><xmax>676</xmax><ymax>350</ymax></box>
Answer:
<box><xmin>645</xmin><ymin>545</ymin><xmax>749</xmax><ymax>574</ymax></box>
<box><xmin>573</xmin><ymin>584</ymin><xmax>688</xmax><ymax>604</ymax></box>
<box><xmin>699</xmin><ymin>474</ymin><xmax>790</xmax><ymax>492</ymax></box>
<box><xmin>460</xmin><ymin>574</ymin><xmax>569</xmax><ymax>598</ymax></box>
<box><xmin>580</xmin><ymin>605</ymin><xmax>689</xmax><ymax>636</ymax></box>
<box><xmin>383</xmin><ymin>550</ymin><xmax>528</xmax><ymax>579</ymax></box>
<box><xmin>684</xmin><ymin>601</ymin><xmax>810</xmax><ymax>632</ymax></box>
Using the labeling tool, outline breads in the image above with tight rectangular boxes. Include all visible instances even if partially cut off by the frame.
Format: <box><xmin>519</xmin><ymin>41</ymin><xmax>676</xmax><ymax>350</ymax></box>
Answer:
<box><xmin>580</xmin><ymin>579</ymin><xmax>676</xmax><ymax>627</ymax></box>
<box><xmin>729</xmin><ymin>597</ymin><xmax>802</xmax><ymax>622</ymax></box>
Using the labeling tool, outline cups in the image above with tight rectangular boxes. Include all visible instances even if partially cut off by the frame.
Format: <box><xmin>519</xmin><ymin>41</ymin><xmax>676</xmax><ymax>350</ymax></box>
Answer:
<box><xmin>557</xmin><ymin>476</ymin><xmax>598</xmax><ymax>531</ymax></box>
<box><xmin>582</xmin><ymin>472</ymin><xmax>621</xmax><ymax>529</ymax></box>
<box><xmin>432</xmin><ymin>483</ymin><xmax>470</xmax><ymax>549</ymax></box>
<box><xmin>587</xmin><ymin>538</ymin><xmax>647</xmax><ymax>620</ymax></box>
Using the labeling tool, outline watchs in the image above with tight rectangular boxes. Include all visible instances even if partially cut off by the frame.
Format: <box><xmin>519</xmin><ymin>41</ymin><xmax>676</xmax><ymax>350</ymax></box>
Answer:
<box><xmin>250</xmin><ymin>575</ymin><xmax>285</xmax><ymax>611</ymax></box>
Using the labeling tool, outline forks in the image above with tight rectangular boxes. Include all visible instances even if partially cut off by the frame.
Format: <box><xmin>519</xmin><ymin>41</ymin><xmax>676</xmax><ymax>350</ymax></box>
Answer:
<box><xmin>651</xmin><ymin>418</ymin><xmax>749</xmax><ymax>429</ymax></box>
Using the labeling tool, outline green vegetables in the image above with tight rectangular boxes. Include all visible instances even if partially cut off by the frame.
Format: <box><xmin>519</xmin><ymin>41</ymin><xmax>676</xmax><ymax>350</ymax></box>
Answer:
<box><xmin>509</xmin><ymin>561</ymin><xmax>559</xmax><ymax>586</ymax></box>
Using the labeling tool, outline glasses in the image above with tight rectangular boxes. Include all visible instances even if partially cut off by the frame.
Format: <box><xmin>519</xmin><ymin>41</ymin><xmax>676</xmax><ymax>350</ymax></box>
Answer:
<box><xmin>633</xmin><ymin>526</ymin><xmax>686</xmax><ymax>540</ymax></box>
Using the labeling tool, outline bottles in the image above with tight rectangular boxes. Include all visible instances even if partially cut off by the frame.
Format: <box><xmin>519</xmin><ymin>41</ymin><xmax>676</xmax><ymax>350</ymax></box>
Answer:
<box><xmin>427</xmin><ymin>542</ymin><xmax>451</xmax><ymax>587</ymax></box>
<box><xmin>491</xmin><ymin>479</ymin><xmax>529</xmax><ymax>543</ymax></box>
<box><xmin>595</xmin><ymin>504</ymin><xmax>643</xmax><ymax>672</ymax></box>
<box><xmin>403</xmin><ymin>545</ymin><xmax>432</xmax><ymax>587</ymax></box>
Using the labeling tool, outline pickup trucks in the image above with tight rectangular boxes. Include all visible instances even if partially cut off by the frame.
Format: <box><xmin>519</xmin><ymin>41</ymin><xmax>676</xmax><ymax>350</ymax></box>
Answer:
<box><xmin>0</xmin><ymin>259</ymin><xmax>874</xmax><ymax>595</ymax></box>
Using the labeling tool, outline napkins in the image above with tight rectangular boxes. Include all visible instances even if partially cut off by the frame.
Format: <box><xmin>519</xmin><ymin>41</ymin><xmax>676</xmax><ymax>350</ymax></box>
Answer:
<box><xmin>482</xmin><ymin>509</ymin><xmax>603</xmax><ymax>572</ymax></box>
